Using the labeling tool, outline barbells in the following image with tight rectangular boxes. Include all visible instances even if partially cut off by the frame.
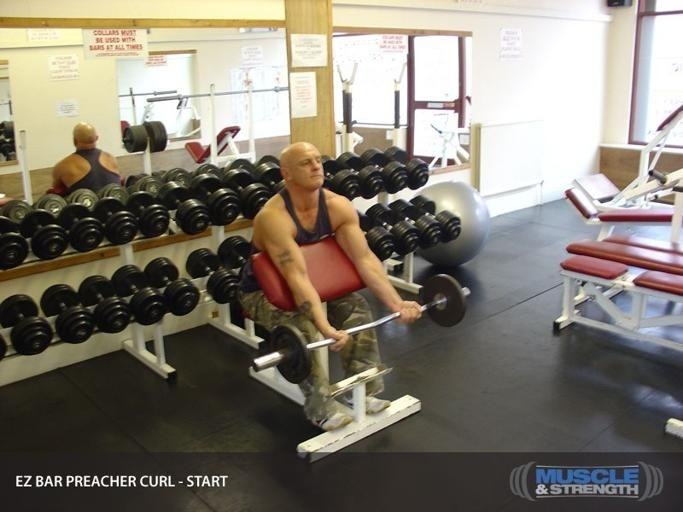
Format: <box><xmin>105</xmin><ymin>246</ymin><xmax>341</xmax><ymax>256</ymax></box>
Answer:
<box><xmin>253</xmin><ymin>274</ymin><xmax>471</xmax><ymax>384</ymax></box>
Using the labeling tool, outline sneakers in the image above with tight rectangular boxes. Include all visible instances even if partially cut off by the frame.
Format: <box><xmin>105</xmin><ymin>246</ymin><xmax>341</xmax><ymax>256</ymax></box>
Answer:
<box><xmin>342</xmin><ymin>395</ymin><xmax>390</xmax><ymax>415</ymax></box>
<box><xmin>311</xmin><ymin>412</ymin><xmax>352</xmax><ymax>431</ymax></box>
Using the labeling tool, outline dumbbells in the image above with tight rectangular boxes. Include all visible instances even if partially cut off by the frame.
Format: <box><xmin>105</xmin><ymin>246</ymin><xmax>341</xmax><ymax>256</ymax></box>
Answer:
<box><xmin>0</xmin><ymin>146</ymin><xmax>461</xmax><ymax>361</ymax></box>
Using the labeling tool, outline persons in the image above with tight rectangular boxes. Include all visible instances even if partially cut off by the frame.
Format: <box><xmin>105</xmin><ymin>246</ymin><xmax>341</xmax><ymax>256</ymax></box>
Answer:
<box><xmin>239</xmin><ymin>142</ymin><xmax>422</xmax><ymax>432</ymax></box>
<box><xmin>49</xmin><ymin>121</ymin><xmax>126</xmax><ymax>199</ymax></box>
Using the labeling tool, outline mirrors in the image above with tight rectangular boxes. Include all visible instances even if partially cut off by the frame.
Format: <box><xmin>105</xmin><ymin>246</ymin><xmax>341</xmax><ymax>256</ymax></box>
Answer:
<box><xmin>0</xmin><ymin>27</ymin><xmax>464</xmax><ymax>214</ymax></box>
